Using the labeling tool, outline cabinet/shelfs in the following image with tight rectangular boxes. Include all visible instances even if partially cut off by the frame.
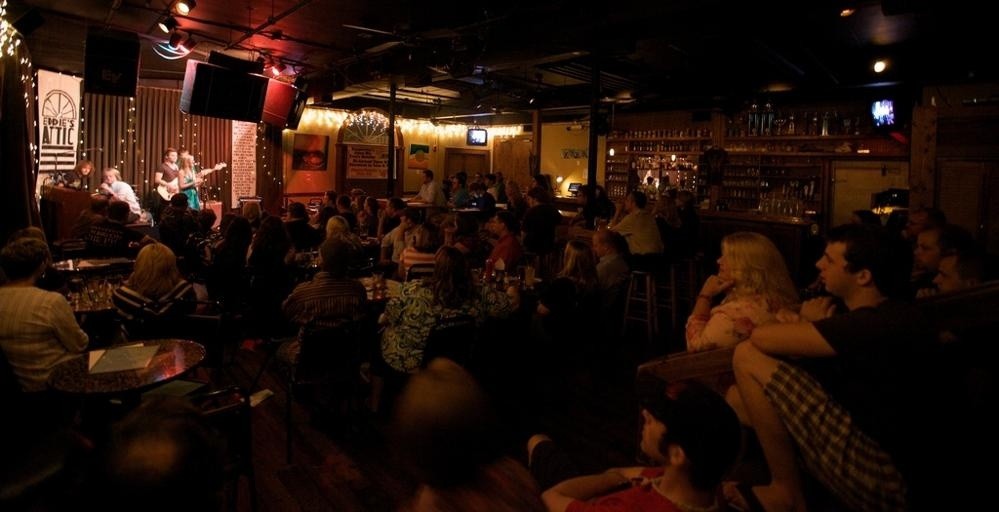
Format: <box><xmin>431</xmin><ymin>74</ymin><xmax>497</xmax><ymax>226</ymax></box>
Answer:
<box><xmin>598</xmin><ymin>132</ymin><xmax>828</xmax><ymax>216</ymax></box>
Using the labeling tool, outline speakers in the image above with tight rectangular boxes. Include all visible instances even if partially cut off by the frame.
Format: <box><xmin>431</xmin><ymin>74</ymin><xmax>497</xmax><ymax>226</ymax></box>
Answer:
<box><xmin>83</xmin><ymin>27</ymin><xmax>141</xmax><ymax>98</ymax></box>
<box><xmin>240</xmin><ymin>199</ymin><xmax>263</xmax><ymax>216</ymax></box>
<box><xmin>205</xmin><ymin>200</ymin><xmax>223</xmax><ymax>230</ymax></box>
<box><xmin>179</xmin><ymin>50</ymin><xmax>268</xmax><ymax>123</ymax></box>
<box><xmin>261</xmin><ymin>77</ymin><xmax>307</xmax><ymax>133</ymax></box>
<box><xmin>40</xmin><ymin>185</ymin><xmax>92</xmax><ymax>241</ymax></box>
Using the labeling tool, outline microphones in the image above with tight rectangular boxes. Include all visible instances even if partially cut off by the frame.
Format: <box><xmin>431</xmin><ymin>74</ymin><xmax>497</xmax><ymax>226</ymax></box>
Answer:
<box><xmin>194</xmin><ymin>162</ymin><xmax>199</xmax><ymax>173</ymax></box>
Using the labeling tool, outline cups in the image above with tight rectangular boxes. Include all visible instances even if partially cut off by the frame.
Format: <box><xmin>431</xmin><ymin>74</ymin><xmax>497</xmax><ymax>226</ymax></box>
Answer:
<box><xmin>372</xmin><ymin>270</ymin><xmax>386</xmax><ymax>290</ymax></box>
<box><xmin>69</xmin><ymin>274</ymin><xmax>120</xmax><ymax>308</ymax></box>
<box><xmin>484</xmin><ymin>256</ymin><xmax>493</xmax><ymax>274</ymax></box>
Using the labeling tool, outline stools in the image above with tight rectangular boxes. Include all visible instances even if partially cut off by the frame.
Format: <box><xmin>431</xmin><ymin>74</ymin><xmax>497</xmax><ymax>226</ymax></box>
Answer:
<box><xmin>620</xmin><ymin>246</ymin><xmax>701</xmax><ymax>341</ymax></box>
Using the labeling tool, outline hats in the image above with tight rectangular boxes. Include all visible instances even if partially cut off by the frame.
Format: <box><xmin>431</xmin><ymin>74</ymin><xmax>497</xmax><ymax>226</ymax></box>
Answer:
<box><xmin>636</xmin><ymin>368</ymin><xmax>744</xmax><ymax>466</ymax></box>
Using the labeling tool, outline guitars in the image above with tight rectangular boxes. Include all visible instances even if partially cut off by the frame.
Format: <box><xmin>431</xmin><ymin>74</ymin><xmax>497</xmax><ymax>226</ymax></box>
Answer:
<box><xmin>196</xmin><ymin>161</ymin><xmax>228</xmax><ymax>178</ymax></box>
<box><xmin>156</xmin><ymin>160</ymin><xmax>179</xmax><ymax>202</ymax></box>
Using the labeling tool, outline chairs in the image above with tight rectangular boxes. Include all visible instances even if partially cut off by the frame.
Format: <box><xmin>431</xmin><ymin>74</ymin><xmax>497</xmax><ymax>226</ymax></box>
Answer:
<box><xmin>175</xmin><ymin>393</ymin><xmax>257</xmax><ymax>511</ymax></box>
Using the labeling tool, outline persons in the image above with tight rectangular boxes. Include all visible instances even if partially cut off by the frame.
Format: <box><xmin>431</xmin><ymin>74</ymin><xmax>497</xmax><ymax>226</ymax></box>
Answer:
<box><xmin>179</xmin><ymin>150</ymin><xmax>206</xmax><ymax>212</ymax></box>
<box><xmin>0</xmin><ymin>167</ymin><xmax>998</xmax><ymax>512</ymax></box>
<box><xmin>407</xmin><ymin>149</ymin><xmax>429</xmax><ymax>172</ymax></box>
<box><xmin>154</xmin><ymin>147</ymin><xmax>179</xmax><ymax>207</ymax></box>
<box><xmin>57</xmin><ymin>159</ymin><xmax>95</xmax><ymax>191</ymax></box>
<box><xmin>293</xmin><ymin>149</ymin><xmax>327</xmax><ymax>171</ymax></box>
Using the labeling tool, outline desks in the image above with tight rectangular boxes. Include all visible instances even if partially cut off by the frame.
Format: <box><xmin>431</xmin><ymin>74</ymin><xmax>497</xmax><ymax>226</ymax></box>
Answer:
<box><xmin>41</xmin><ymin>191</ymin><xmax>583</xmax><ymax>396</ymax></box>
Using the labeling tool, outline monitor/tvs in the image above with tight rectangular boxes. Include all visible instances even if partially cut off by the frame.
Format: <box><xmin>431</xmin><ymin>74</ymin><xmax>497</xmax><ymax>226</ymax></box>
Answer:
<box><xmin>870</xmin><ymin>99</ymin><xmax>897</xmax><ymax>129</ymax></box>
<box><xmin>466</xmin><ymin>129</ymin><xmax>488</xmax><ymax>146</ymax></box>
<box><xmin>568</xmin><ymin>183</ymin><xmax>582</xmax><ymax>197</ymax></box>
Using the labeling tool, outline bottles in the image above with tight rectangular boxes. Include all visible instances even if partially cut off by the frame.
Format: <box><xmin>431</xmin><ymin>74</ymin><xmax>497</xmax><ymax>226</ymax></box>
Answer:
<box><xmin>603</xmin><ymin>126</ymin><xmax>712</xmax><ymax>198</ymax></box>
<box><xmin>718</xmin><ymin>158</ymin><xmax>811</xmax><ymax>219</ymax></box>
<box><xmin>524</xmin><ymin>264</ymin><xmax>536</xmax><ymax>287</ymax></box>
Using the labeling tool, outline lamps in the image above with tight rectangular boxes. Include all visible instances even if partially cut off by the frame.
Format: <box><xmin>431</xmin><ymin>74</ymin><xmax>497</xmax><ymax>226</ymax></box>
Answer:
<box><xmin>157</xmin><ymin>0</ymin><xmax>198</xmax><ymax>49</ymax></box>
